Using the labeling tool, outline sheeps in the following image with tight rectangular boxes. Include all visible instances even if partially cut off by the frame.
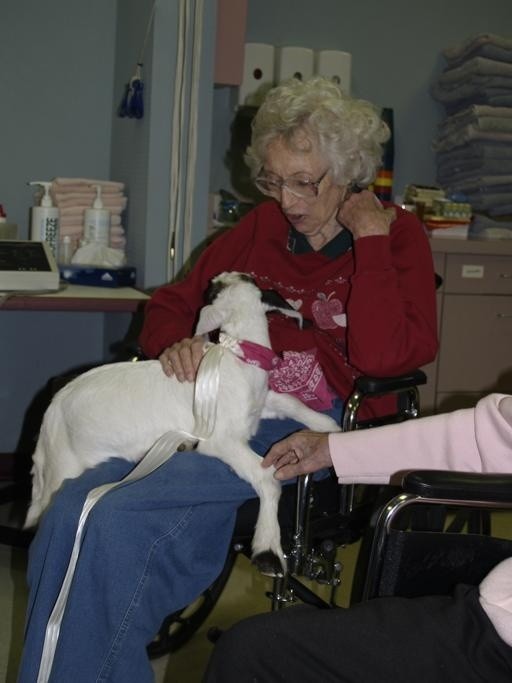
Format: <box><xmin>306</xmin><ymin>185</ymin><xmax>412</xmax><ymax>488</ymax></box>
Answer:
<box><xmin>15</xmin><ymin>270</ymin><xmax>342</xmax><ymax>580</ymax></box>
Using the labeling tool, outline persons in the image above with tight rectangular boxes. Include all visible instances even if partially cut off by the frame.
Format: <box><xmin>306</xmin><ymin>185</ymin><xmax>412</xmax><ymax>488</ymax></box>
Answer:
<box><xmin>194</xmin><ymin>390</ymin><xmax>511</xmax><ymax>683</ymax></box>
<box><xmin>15</xmin><ymin>72</ymin><xmax>442</xmax><ymax>683</ymax></box>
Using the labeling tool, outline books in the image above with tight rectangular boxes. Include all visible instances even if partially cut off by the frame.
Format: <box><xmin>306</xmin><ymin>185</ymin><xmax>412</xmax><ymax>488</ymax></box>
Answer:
<box><xmin>399</xmin><ymin>183</ymin><xmax>473</xmax><ymax>238</ymax></box>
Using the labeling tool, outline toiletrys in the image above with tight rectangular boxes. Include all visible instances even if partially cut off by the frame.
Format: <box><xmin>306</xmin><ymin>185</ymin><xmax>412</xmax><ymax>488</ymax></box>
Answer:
<box><xmin>28</xmin><ymin>182</ymin><xmax>59</xmax><ymax>264</ymax></box>
<box><xmin>81</xmin><ymin>183</ymin><xmax>110</xmax><ymax>249</ymax></box>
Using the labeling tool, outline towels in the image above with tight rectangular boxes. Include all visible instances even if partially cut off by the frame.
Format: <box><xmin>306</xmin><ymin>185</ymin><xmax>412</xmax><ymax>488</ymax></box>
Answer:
<box><xmin>436</xmin><ymin>28</ymin><xmax>512</xmax><ymax>228</ymax></box>
<box><xmin>34</xmin><ymin>173</ymin><xmax>126</xmax><ymax>253</ymax></box>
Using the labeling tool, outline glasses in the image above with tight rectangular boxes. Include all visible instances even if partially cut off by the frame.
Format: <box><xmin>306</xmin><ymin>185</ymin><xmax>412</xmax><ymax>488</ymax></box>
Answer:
<box><xmin>254</xmin><ymin>166</ymin><xmax>333</xmax><ymax>199</ymax></box>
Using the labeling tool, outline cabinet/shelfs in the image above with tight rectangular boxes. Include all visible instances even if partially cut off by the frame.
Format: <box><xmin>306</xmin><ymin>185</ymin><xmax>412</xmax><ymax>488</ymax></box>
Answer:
<box><xmin>419</xmin><ymin>228</ymin><xmax>512</xmax><ymax>418</ymax></box>
